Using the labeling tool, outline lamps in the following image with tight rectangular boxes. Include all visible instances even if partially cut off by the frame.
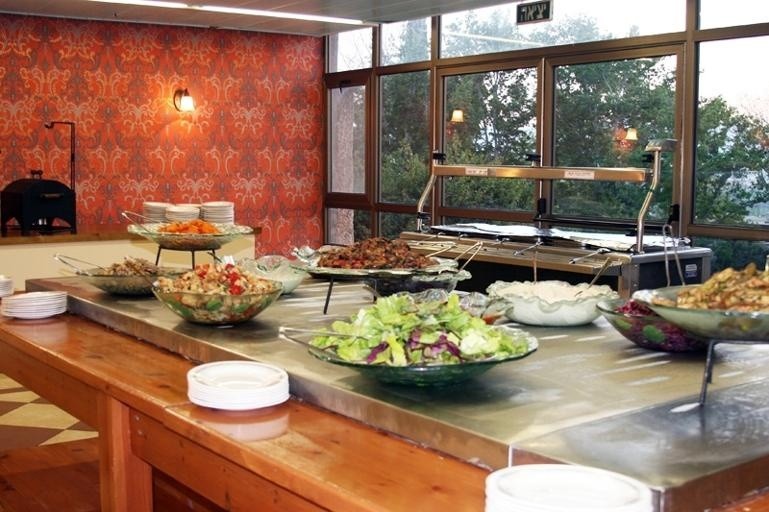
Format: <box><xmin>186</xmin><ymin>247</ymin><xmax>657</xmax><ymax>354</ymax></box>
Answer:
<box><xmin>173</xmin><ymin>88</ymin><xmax>195</xmax><ymax>113</ymax></box>
<box><xmin>450</xmin><ymin>108</ymin><xmax>464</xmax><ymax>123</ymax></box>
<box><xmin>625</xmin><ymin>127</ymin><xmax>638</xmax><ymax>140</ymax></box>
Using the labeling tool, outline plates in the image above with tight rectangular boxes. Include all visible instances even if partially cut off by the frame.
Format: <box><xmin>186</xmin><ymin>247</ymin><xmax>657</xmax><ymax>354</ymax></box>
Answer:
<box><xmin>5</xmin><ymin>324</ymin><xmax>67</xmax><ymax>344</ymax></box>
<box><xmin>632</xmin><ymin>285</ymin><xmax>768</xmax><ymax>339</ymax></box>
<box><xmin>486</xmin><ymin>279</ymin><xmax>618</xmax><ymax>329</ymax></box>
<box><xmin>148</xmin><ymin>276</ymin><xmax>283</xmax><ymax>329</ymax></box>
<box><xmin>241</xmin><ymin>261</ymin><xmax>311</xmax><ymax>296</ymax></box>
<box><xmin>276</xmin><ymin>316</ymin><xmax>539</xmax><ymax>389</ymax></box>
<box><xmin>484</xmin><ymin>464</ymin><xmax>654</xmax><ymax>512</ymax></box>
<box><xmin>1</xmin><ymin>276</ymin><xmax>14</xmax><ymax>298</ymax></box>
<box><xmin>78</xmin><ymin>267</ymin><xmax>189</xmax><ymax>299</ymax></box>
<box><xmin>292</xmin><ymin>251</ymin><xmax>462</xmax><ymax>284</ymax></box>
<box><xmin>186</xmin><ymin>361</ymin><xmax>291</xmax><ymax>409</ymax></box>
<box><xmin>127</xmin><ymin>223</ymin><xmax>253</xmax><ymax>251</ymax></box>
<box><xmin>198</xmin><ymin>416</ymin><xmax>289</xmax><ymax>443</ymax></box>
<box><xmin>597</xmin><ymin>297</ymin><xmax>693</xmax><ymax>352</ymax></box>
<box><xmin>358</xmin><ymin>267</ymin><xmax>472</xmax><ymax>298</ymax></box>
<box><xmin>142</xmin><ymin>201</ymin><xmax>236</xmax><ymax>224</ymax></box>
<box><xmin>0</xmin><ymin>291</ymin><xmax>66</xmax><ymax>319</ymax></box>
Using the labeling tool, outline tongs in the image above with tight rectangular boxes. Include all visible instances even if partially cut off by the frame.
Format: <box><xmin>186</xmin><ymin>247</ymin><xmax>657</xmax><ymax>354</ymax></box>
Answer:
<box><xmin>53</xmin><ymin>252</ymin><xmax>113</xmax><ymax>278</ymax></box>
<box><xmin>122</xmin><ymin>209</ymin><xmax>167</xmax><ymax>238</ymax></box>
<box><xmin>662</xmin><ymin>225</ymin><xmax>687</xmax><ymax>287</ymax></box>
